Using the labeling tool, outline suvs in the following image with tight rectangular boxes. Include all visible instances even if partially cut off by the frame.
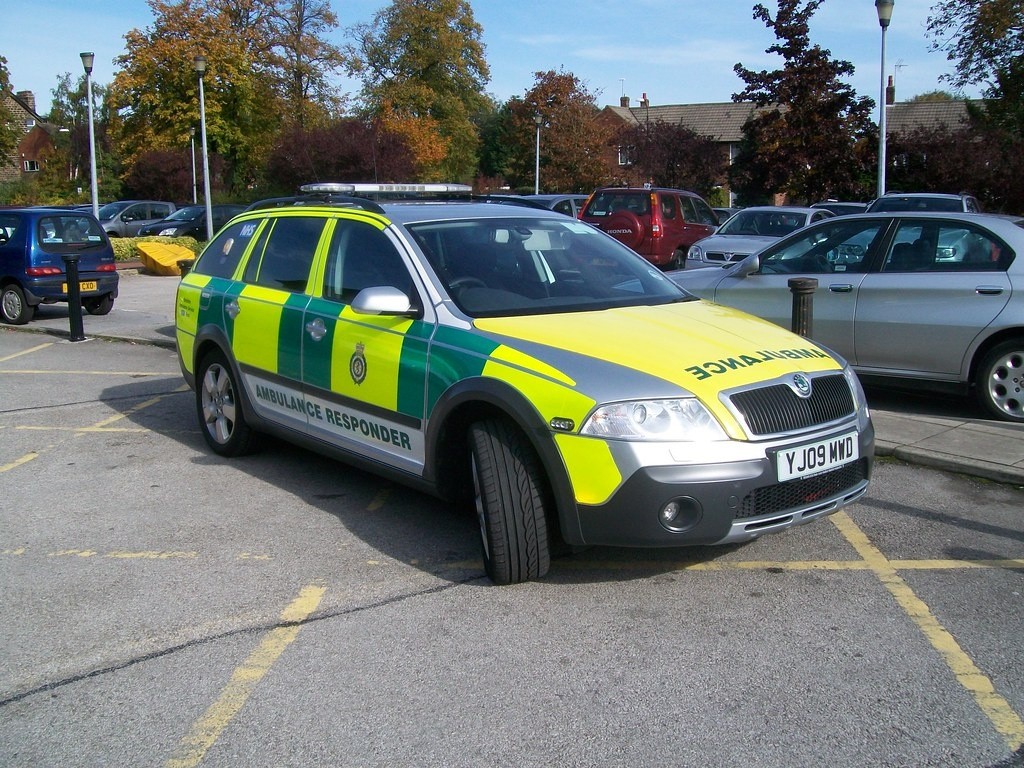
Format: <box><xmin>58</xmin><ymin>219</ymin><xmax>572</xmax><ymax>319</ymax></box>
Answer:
<box><xmin>834</xmin><ymin>189</ymin><xmax>993</xmax><ymax>264</ymax></box>
<box><xmin>576</xmin><ymin>185</ymin><xmax>722</xmax><ymax>275</ymax></box>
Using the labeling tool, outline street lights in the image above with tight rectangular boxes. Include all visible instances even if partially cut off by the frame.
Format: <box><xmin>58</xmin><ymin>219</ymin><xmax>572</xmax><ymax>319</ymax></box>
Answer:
<box><xmin>533</xmin><ymin>113</ymin><xmax>544</xmax><ymax>195</ymax></box>
<box><xmin>79</xmin><ymin>50</ymin><xmax>100</xmax><ymax>223</ymax></box>
<box><xmin>872</xmin><ymin>1</ymin><xmax>897</xmax><ymax>199</ymax></box>
<box><xmin>191</xmin><ymin>51</ymin><xmax>215</xmax><ymax>242</ymax></box>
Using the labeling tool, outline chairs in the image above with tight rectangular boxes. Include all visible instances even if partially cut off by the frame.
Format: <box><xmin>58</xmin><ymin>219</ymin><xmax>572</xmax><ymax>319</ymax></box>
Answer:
<box><xmin>55</xmin><ymin>221</ymin><xmax>88</xmax><ymax>242</ymax></box>
<box><xmin>0</xmin><ymin>225</ymin><xmax>9</xmax><ymax>244</ymax></box>
<box><xmin>890</xmin><ymin>239</ymin><xmax>932</xmax><ymax>270</ymax></box>
<box><xmin>450</xmin><ymin>243</ymin><xmax>498</xmax><ymax>289</ymax></box>
<box><xmin>614</xmin><ymin>201</ymin><xmax>629</xmax><ymax>211</ymax></box>
<box><xmin>41</xmin><ymin>226</ymin><xmax>49</xmax><ymax>238</ymax></box>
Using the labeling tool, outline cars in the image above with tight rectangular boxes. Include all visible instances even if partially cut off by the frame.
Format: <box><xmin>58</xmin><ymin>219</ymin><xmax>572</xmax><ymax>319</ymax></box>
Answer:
<box><xmin>612</xmin><ymin>210</ymin><xmax>1024</xmax><ymax>424</ymax></box>
<box><xmin>173</xmin><ymin>180</ymin><xmax>876</xmax><ymax>588</ymax></box>
<box><xmin>712</xmin><ymin>207</ymin><xmax>741</xmax><ymax>228</ymax></box>
<box><xmin>809</xmin><ymin>201</ymin><xmax>869</xmax><ymax>215</ymax></box>
<box><xmin>7</xmin><ymin>198</ymin><xmax>251</xmax><ymax>242</ymax></box>
<box><xmin>684</xmin><ymin>204</ymin><xmax>839</xmax><ymax>269</ymax></box>
<box><xmin>500</xmin><ymin>193</ymin><xmax>614</xmax><ymax>219</ymax></box>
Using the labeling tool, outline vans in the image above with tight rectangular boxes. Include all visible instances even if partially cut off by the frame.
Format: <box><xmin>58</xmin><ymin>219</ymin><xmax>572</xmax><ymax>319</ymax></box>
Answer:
<box><xmin>0</xmin><ymin>205</ymin><xmax>120</xmax><ymax>327</ymax></box>
